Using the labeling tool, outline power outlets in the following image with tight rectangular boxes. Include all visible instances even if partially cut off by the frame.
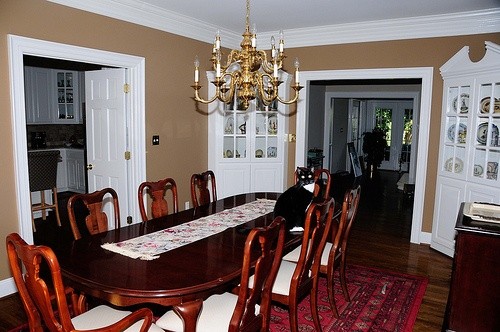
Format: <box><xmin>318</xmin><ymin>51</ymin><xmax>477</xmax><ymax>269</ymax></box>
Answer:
<box><xmin>184</xmin><ymin>201</ymin><xmax>190</xmax><ymax>210</ymax></box>
<box><xmin>152</xmin><ymin>134</ymin><xmax>159</xmax><ymax>145</ymax></box>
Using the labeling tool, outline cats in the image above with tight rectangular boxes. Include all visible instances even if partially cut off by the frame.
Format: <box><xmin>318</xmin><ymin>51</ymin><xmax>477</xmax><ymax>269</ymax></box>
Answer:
<box><xmin>273</xmin><ymin>166</ymin><xmax>316</xmax><ymax>232</ymax></box>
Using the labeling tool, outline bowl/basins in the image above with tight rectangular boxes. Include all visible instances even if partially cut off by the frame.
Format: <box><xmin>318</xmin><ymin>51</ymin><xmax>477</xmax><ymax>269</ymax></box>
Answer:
<box><xmin>65</xmin><ymin>143</ymin><xmax>72</xmax><ymax>146</ymax></box>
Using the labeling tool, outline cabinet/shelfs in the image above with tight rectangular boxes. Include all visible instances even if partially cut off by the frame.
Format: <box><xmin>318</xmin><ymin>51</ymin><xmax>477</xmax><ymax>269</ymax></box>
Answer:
<box><xmin>206</xmin><ymin>58</ymin><xmax>294</xmax><ymax>203</ymax></box>
<box><xmin>430</xmin><ymin>40</ymin><xmax>500</xmax><ymax>258</ymax></box>
<box><xmin>28</xmin><ymin>148</ymin><xmax>86</xmax><ymax>219</ymax></box>
<box><xmin>23</xmin><ymin>66</ymin><xmax>86</xmax><ymax>125</ymax></box>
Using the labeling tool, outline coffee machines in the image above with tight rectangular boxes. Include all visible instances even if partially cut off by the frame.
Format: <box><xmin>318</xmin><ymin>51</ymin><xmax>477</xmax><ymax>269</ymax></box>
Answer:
<box><xmin>30</xmin><ymin>130</ymin><xmax>47</xmax><ymax>149</ymax></box>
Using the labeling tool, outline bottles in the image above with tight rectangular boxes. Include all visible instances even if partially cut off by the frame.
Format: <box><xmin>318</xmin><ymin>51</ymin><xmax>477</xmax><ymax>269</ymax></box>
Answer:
<box><xmin>57</xmin><ymin>79</ymin><xmax>73</xmax><ymax>103</ymax></box>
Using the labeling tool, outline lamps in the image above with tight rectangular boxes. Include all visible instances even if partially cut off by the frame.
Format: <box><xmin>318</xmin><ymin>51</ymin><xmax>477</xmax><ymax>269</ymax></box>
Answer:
<box><xmin>189</xmin><ymin>0</ymin><xmax>305</xmax><ymax>109</ymax></box>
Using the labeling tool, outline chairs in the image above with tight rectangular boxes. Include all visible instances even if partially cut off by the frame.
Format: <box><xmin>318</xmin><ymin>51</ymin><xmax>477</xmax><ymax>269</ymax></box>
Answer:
<box><xmin>67</xmin><ymin>188</ymin><xmax>120</xmax><ymax>311</ymax></box>
<box><xmin>190</xmin><ymin>170</ymin><xmax>217</xmax><ymax>208</ymax></box>
<box><xmin>6</xmin><ymin>233</ymin><xmax>165</xmax><ymax>332</ymax></box>
<box><xmin>155</xmin><ymin>215</ymin><xmax>286</xmax><ymax>332</ymax></box>
<box><xmin>307</xmin><ymin>148</ymin><xmax>323</xmax><ymax>179</ymax></box>
<box><xmin>229</xmin><ymin>197</ymin><xmax>336</xmax><ymax>332</ymax></box>
<box><xmin>293</xmin><ymin>167</ymin><xmax>331</xmax><ymax>201</ymax></box>
<box><xmin>281</xmin><ymin>185</ymin><xmax>361</xmax><ymax>320</ymax></box>
<box><xmin>138</xmin><ymin>178</ymin><xmax>179</xmax><ymax>222</ymax></box>
<box><xmin>27</xmin><ymin>149</ymin><xmax>63</xmax><ymax>232</ymax></box>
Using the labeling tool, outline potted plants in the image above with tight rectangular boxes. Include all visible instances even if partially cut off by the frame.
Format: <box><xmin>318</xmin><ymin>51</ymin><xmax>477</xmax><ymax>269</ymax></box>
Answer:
<box><xmin>361</xmin><ymin>126</ymin><xmax>390</xmax><ymax>179</ymax></box>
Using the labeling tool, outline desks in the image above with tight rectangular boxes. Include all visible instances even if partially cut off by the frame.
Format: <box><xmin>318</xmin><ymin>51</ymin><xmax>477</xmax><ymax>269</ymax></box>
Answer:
<box><xmin>441</xmin><ymin>201</ymin><xmax>500</xmax><ymax>332</ymax></box>
<box><xmin>43</xmin><ymin>192</ymin><xmax>350</xmax><ymax>332</ymax></box>
<box><xmin>306</xmin><ymin>154</ymin><xmax>326</xmax><ymax>180</ymax></box>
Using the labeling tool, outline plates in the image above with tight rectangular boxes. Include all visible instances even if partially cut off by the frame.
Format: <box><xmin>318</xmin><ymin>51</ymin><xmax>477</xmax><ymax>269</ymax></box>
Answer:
<box><xmin>225</xmin><ymin>112</ymin><xmax>278</xmax><ymax>158</ymax></box>
<box><xmin>444</xmin><ymin>93</ymin><xmax>500</xmax><ymax>182</ymax></box>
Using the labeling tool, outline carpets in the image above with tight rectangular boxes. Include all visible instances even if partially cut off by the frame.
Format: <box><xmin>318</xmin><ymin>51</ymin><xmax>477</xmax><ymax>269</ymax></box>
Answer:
<box><xmin>267</xmin><ymin>263</ymin><xmax>430</xmax><ymax>332</ymax></box>
